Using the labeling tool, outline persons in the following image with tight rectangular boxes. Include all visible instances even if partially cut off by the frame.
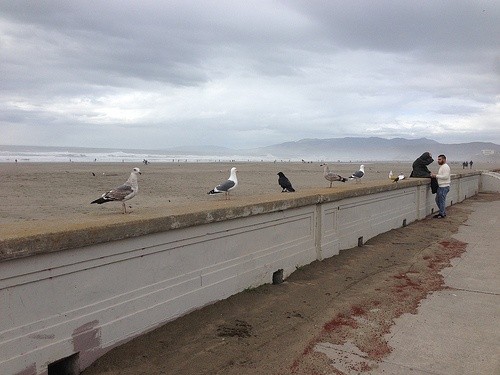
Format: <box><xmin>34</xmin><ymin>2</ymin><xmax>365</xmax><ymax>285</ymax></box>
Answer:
<box><xmin>430</xmin><ymin>154</ymin><xmax>451</xmax><ymax>219</ymax></box>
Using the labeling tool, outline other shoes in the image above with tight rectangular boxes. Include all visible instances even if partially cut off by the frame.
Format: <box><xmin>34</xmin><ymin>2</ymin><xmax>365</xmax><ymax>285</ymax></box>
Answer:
<box><xmin>433</xmin><ymin>213</ymin><xmax>444</xmax><ymax>218</ymax></box>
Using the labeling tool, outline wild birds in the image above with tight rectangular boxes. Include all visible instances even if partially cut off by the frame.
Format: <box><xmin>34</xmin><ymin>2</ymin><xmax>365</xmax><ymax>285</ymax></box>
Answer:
<box><xmin>15</xmin><ymin>159</ymin><xmax>17</xmax><ymax>162</ymax></box>
<box><xmin>388</xmin><ymin>169</ymin><xmax>394</xmax><ymax>180</ymax></box>
<box><xmin>206</xmin><ymin>166</ymin><xmax>239</xmax><ymax>201</ymax></box>
<box><xmin>276</xmin><ymin>172</ymin><xmax>295</xmax><ymax>193</ymax></box>
<box><xmin>89</xmin><ymin>167</ymin><xmax>142</xmax><ymax>214</ymax></box>
<box><xmin>319</xmin><ymin>162</ymin><xmax>348</xmax><ymax>188</ymax></box>
<box><xmin>392</xmin><ymin>172</ymin><xmax>407</xmax><ymax>183</ymax></box>
<box><xmin>143</xmin><ymin>159</ymin><xmax>149</xmax><ymax>165</ymax></box>
<box><xmin>94</xmin><ymin>158</ymin><xmax>96</xmax><ymax>161</ymax></box>
<box><xmin>170</xmin><ymin>159</ymin><xmax>324</xmax><ymax>165</ymax></box>
<box><xmin>70</xmin><ymin>159</ymin><xmax>72</xmax><ymax>162</ymax></box>
<box><xmin>349</xmin><ymin>164</ymin><xmax>365</xmax><ymax>183</ymax></box>
